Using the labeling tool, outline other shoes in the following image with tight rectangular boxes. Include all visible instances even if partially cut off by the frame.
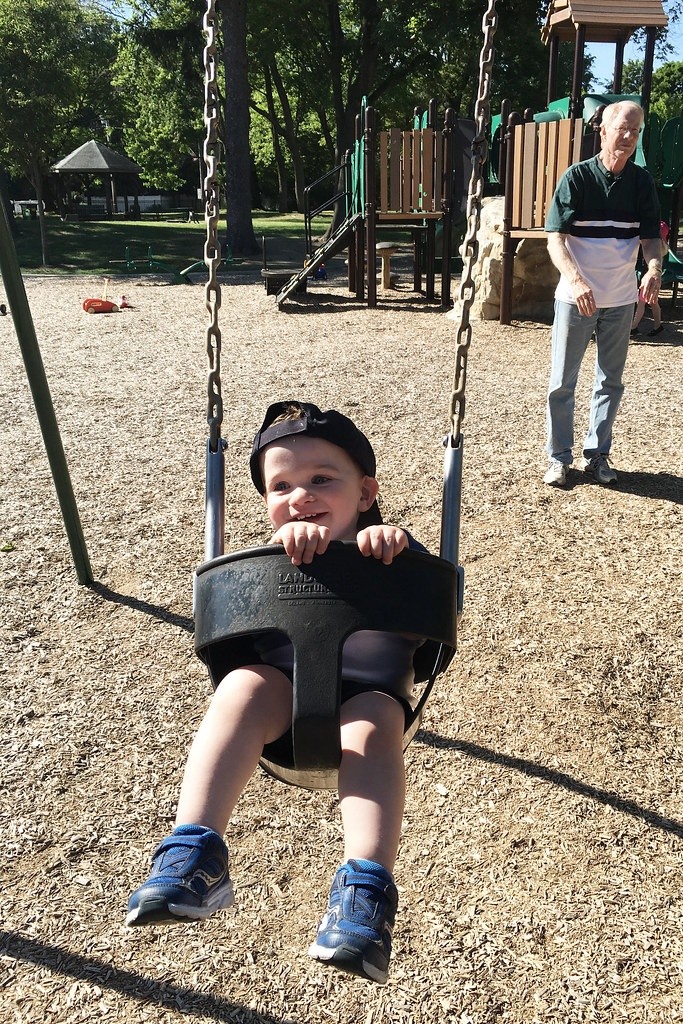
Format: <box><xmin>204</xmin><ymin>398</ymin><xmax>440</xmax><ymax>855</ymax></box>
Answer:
<box><xmin>629</xmin><ymin>328</ymin><xmax>638</xmax><ymax>335</ymax></box>
<box><xmin>648</xmin><ymin>325</ymin><xmax>664</xmax><ymax>337</ymax></box>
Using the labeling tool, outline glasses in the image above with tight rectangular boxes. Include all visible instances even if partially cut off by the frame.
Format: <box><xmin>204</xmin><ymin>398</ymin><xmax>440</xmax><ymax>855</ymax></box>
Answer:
<box><xmin>606</xmin><ymin>126</ymin><xmax>641</xmax><ymax>136</ymax></box>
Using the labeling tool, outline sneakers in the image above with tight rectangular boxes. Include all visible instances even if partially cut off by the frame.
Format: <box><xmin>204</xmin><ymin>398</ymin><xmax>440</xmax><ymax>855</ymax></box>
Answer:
<box><xmin>308</xmin><ymin>857</ymin><xmax>398</xmax><ymax>983</ymax></box>
<box><xmin>126</xmin><ymin>824</ymin><xmax>234</xmax><ymax>926</ymax></box>
<box><xmin>543</xmin><ymin>461</ymin><xmax>570</xmax><ymax>486</ymax></box>
<box><xmin>582</xmin><ymin>453</ymin><xmax>619</xmax><ymax>486</ymax></box>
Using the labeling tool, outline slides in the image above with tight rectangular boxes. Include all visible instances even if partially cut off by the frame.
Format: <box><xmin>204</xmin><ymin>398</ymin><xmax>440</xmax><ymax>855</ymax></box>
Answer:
<box><xmin>636</xmin><ymin>246</ymin><xmax>683</xmax><ymax>291</ymax></box>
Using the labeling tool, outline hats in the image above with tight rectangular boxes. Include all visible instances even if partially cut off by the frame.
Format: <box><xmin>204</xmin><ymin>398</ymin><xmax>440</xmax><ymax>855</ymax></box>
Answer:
<box><xmin>249</xmin><ymin>401</ymin><xmax>382</xmax><ymax>531</ymax></box>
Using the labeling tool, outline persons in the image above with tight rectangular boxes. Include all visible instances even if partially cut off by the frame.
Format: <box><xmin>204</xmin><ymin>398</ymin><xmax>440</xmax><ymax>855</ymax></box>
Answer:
<box><xmin>126</xmin><ymin>404</ymin><xmax>429</xmax><ymax>984</ymax></box>
<box><xmin>543</xmin><ymin>100</ymin><xmax>667</xmax><ymax>485</ymax></box>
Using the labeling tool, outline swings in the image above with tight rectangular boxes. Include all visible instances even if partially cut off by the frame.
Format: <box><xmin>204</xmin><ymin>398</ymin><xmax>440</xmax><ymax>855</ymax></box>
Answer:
<box><xmin>191</xmin><ymin>0</ymin><xmax>500</xmax><ymax>791</ymax></box>
